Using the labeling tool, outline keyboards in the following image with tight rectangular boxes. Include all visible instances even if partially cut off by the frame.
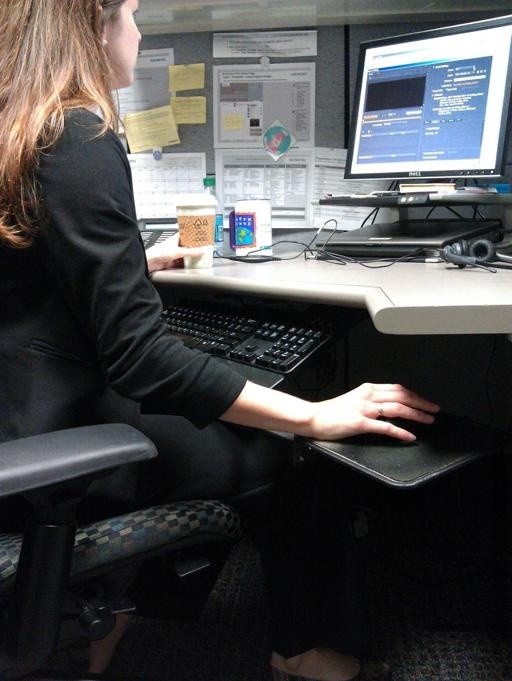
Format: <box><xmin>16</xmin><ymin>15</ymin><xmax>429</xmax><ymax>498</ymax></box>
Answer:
<box><xmin>160</xmin><ymin>298</ymin><xmax>332</xmax><ymax>376</ymax></box>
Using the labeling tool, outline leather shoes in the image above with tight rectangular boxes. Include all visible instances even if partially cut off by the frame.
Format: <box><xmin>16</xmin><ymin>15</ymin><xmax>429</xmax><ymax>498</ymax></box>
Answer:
<box><xmin>269</xmin><ymin>656</ymin><xmax>391</xmax><ymax>681</ymax></box>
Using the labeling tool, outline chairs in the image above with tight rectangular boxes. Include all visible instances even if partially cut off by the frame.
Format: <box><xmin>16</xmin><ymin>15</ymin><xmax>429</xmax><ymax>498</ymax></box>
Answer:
<box><xmin>0</xmin><ymin>422</ymin><xmax>241</xmax><ymax>681</ymax></box>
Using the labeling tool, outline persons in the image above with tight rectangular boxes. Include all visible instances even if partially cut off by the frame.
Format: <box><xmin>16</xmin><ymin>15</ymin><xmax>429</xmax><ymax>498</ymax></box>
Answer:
<box><xmin>0</xmin><ymin>0</ymin><xmax>444</xmax><ymax>679</ymax></box>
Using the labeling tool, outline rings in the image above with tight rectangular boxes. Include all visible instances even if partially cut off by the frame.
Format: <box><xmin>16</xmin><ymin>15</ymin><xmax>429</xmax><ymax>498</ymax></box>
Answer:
<box><xmin>375</xmin><ymin>402</ymin><xmax>385</xmax><ymax>417</ymax></box>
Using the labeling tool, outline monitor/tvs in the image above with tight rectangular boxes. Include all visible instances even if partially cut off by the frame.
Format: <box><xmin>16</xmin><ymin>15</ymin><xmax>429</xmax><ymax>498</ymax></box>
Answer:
<box><xmin>344</xmin><ymin>15</ymin><xmax>512</xmax><ymax>197</ymax></box>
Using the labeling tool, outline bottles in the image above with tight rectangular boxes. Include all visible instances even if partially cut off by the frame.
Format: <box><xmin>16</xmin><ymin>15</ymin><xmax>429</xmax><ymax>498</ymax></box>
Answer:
<box><xmin>202</xmin><ymin>177</ymin><xmax>224</xmax><ymax>259</ymax></box>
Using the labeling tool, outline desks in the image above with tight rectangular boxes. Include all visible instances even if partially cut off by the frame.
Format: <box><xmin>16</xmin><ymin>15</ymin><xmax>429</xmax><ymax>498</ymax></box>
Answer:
<box><xmin>150</xmin><ymin>252</ymin><xmax>512</xmax><ymax>336</ymax></box>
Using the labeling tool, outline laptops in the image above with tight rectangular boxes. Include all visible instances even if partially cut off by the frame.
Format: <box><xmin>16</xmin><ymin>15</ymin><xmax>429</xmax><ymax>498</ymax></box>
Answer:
<box><xmin>315</xmin><ymin>221</ymin><xmax>504</xmax><ymax>263</ymax></box>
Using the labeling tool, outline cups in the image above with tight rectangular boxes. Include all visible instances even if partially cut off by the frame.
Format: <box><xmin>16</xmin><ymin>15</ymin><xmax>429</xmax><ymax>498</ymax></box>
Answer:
<box><xmin>175</xmin><ymin>193</ymin><xmax>219</xmax><ymax>271</ymax></box>
<box><xmin>227</xmin><ymin>192</ymin><xmax>273</xmax><ymax>256</ymax></box>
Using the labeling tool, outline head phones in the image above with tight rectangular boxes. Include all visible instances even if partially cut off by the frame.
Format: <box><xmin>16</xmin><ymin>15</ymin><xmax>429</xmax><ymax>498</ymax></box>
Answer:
<box><xmin>440</xmin><ymin>238</ymin><xmax>512</xmax><ymax>274</ymax></box>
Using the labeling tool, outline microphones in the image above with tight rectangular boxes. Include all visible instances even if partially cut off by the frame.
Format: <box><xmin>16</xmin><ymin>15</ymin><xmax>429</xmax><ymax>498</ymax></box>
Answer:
<box><xmin>471</xmin><ymin>263</ymin><xmax>497</xmax><ymax>273</ymax></box>
